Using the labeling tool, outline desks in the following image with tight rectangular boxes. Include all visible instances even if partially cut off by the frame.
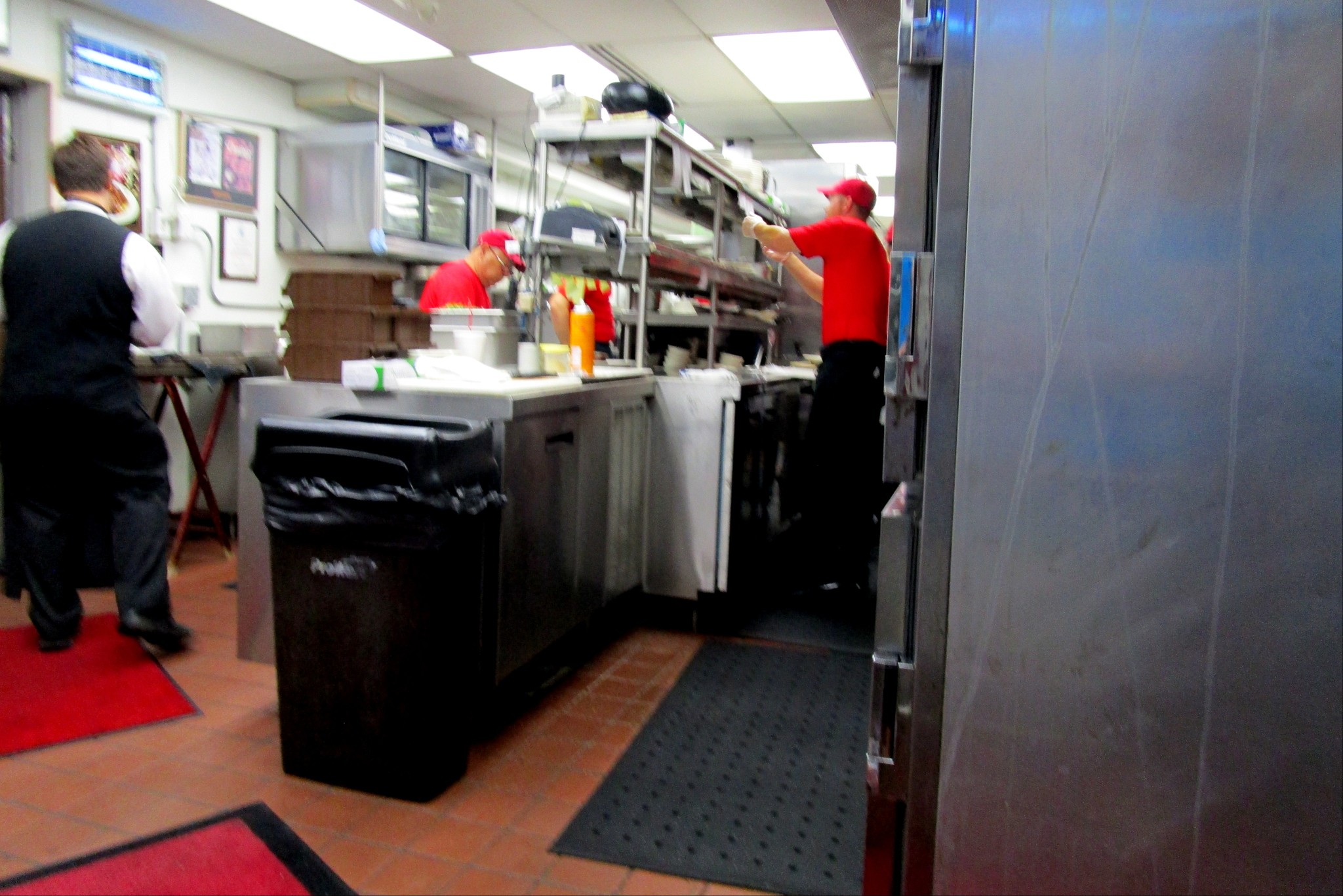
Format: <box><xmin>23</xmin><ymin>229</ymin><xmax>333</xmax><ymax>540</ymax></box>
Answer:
<box><xmin>126</xmin><ymin>355</ymin><xmax>281</xmax><ymax>576</ymax></box>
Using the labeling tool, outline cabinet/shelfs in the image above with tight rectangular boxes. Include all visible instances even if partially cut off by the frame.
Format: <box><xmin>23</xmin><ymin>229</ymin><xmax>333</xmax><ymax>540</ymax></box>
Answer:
<box><xmin>292</xmin><ymin>124</ymin><xmax>497</xmax><ymax>262</ymax></box>
<box><xmin>530</xmin><ymin>114</ymin><xmax>789</xmax><ymax>370</ymax></box>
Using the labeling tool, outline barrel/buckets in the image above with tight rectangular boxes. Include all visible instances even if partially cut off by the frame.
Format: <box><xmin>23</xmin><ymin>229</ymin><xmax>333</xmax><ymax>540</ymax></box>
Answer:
<box><xmin>200</xmin><ymin>323</ymin><xmax>243</xmax><ymax>356</ymax></box>
<box><xmin>243</xmin><ymin>325</ymin><xmax>276</xmax><ymax>358</ymax></box>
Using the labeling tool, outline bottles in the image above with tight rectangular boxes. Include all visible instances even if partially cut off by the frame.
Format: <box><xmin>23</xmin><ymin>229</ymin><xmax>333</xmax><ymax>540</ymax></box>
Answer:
<box><xmin>571</xmin><ymin>300</ymin><xmax>595</xmax><ymax>377</ymax></box>
<box><xmin>799</xmin><ymin>383</ymin><xmax>814</xmax><ymax>420</ymax></box>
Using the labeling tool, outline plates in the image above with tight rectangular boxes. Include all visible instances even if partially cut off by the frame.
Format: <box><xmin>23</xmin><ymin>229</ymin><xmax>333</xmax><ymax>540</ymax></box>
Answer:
<box><xmin>705</xmin><ymin>150</ymin><xmax>763</xmax><ymax>193</ymax></box>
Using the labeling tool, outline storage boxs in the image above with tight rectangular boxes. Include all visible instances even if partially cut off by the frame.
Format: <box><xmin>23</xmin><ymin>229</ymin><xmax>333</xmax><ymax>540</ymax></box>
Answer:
<box><xmin>198</xmin><ymin>326</ymin><xmax>277</xmax><ymax>352</ymax></box>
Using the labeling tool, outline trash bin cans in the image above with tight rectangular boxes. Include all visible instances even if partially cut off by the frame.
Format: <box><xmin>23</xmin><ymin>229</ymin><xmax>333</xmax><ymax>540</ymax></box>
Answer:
<box><xmin>251</xmin><ymin>408</ymin><xmax>506</xmax><ymax>803</ymax></box>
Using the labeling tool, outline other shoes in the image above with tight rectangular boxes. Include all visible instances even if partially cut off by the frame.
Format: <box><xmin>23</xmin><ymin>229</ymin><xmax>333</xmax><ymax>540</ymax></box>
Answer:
<box><xmin>122</xmin><ymin>611</ymin><xmax>189</xmax><ymax>645</ymax></box>
<box><xmin>37</xmin><ymin>636</ymin><xmax>72</xmax><ymax>650</ymax></box>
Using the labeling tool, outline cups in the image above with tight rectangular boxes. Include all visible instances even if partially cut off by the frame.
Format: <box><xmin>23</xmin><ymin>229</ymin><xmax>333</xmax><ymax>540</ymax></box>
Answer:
<box><xmin>517</xmin><ymin>342</ymin><xmax>539</xmax><ymax>375</ymax></box>
<box><xmin>454</xmin><ymin>331</ymin><xmax>487</xmax><ymax>363</ymax></box>
<box><xmin>518</xmin><ymin>292</ymin><xmax>533</xmax><ymax>312</ymax></box>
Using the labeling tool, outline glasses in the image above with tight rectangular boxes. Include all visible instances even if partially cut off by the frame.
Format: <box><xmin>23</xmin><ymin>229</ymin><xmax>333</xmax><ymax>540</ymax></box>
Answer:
<box><xmin>488</xmin><ymin>246</ymin><xmax>513</xmax><ymax>276</ymax></box>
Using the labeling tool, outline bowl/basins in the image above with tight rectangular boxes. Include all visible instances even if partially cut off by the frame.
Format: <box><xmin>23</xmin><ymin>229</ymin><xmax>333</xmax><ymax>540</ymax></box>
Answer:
<box><xmin>790</xmin><ymin>353</ymin><xmax>823</xmax><ymax>369</ymax></box>
<box><xmin>408</xmin><ymin>349</ymin><xmax>456</xmax><ymax>358</ymax></box>
<box><xmin>714</xmin><ymin>352</ymin><xmax>743</xmax><ymax>372</ymax></box>
<box><xmin>537</xmin><ymin>344</ymin><xmax>570</xmax><ymax>376</ymax></box>
<box><xmin>594</xmin><ymin>351</ymin><xmax>635</xmax><ymax>366</ymax></box>
<box><xmin>664</xmin><ymin>345</ymin><xmax>689</xmax><ymax>377</ymax></box>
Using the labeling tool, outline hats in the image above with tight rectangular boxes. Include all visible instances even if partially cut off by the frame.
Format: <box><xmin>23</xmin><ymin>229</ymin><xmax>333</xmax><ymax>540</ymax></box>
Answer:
<box><xmin>479</xmin><ymin>229</ymin><xmax>526</xmax><ymax>272</ymax></box>
<box><xmin>818</xmin><ymin>178</ymin><xmax>876</xmax><ymax>211</ymax></box>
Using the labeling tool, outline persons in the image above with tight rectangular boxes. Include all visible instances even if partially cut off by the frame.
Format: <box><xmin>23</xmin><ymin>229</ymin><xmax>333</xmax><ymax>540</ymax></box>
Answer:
<box><xmin>421</xmin><ymin>228</ymin><xmax>530</xmax><ymax>315</ymax></box>
<box><xmin>740</xmin><ymin>179</ymin><xmax>895</xmax><ymax>618</ymax></box>
<box><xmin>1</xmin><ymin>139</ymin><xmax>192</xmax><ymax>651</ymax></box>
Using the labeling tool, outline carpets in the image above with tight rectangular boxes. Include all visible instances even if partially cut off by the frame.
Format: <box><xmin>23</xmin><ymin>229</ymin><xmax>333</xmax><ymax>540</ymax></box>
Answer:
<box><xmin>0</xmin><ymin>797</ymin><xmax>359</xmax><ymax>896</ymax></box>
<box><xmin>547</xmin><ymin>641</ymin><xmax>874</xmax><ymax>896</ymax></box>
<box><xmin>748</xmin><ymin>606</ymin><xmax>875</xmax><ymax>657</ymax></box>
<box><xmin>0</xmin><ymin>611</ymin><xmax>201</xmax><ymax>763</ymax></box>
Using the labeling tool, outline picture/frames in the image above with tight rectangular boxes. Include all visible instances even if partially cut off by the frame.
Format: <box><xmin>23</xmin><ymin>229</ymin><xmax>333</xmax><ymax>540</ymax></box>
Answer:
<box><xmin>74</xmin><ymin>131</ymin><xmax>142</xmax><ymax>235</ymax></box>
